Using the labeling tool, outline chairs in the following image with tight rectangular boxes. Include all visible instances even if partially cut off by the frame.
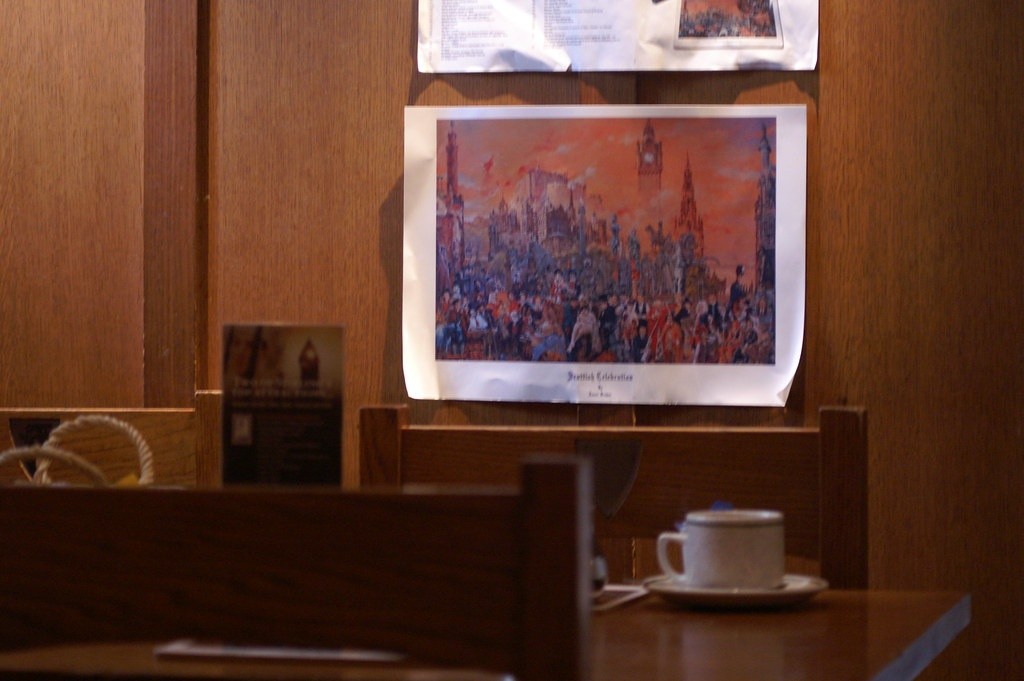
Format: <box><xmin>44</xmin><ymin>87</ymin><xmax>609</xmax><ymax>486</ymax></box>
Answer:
<box><xmin>0</xmin><ymin>453</ymin><xmax>589</xmax><ymax>681</ymax></box>
<box><xmin>358</xmin><ymin>405</ymin><xmax>869</xmax><ymax>589</ymax></box>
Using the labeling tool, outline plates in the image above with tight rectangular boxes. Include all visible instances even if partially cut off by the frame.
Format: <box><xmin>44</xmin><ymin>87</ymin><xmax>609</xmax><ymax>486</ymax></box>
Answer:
<box><xmin>649</xmin><ymin>577</ymin><xmax>828</xmax><ymax>606</ymax></box>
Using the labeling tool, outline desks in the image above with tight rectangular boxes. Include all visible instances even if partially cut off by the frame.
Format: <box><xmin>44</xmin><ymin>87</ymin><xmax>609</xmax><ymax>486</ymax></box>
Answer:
<box><xmin>1</xmin><ymin>589</ymin><xmax>973</xmax><ymax>681</ymax></box>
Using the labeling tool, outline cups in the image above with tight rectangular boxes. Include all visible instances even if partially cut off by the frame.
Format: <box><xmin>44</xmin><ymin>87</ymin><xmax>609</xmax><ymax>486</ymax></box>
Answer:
<box><xmin>655</xmin><ymin>508</ymin><xmax>785</xmax><ymax>591</ymax></box>
